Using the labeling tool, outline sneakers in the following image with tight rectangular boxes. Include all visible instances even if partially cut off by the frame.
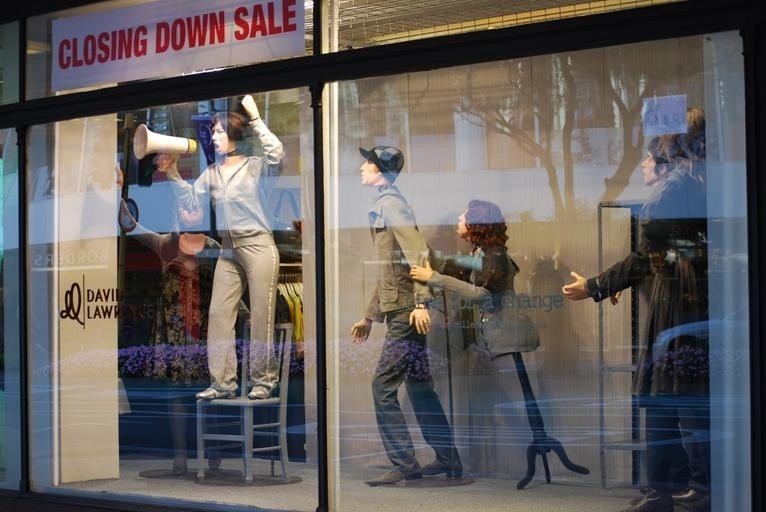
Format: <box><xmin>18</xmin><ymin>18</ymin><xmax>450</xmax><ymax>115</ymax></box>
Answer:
<box><xmin>195</xmin><ymin>387</ymin><xmax>237</xmax><ymax>400</ymax></box>
<box><xmin>248</xmin><ymin>385</ymin><xmax>272</xmax><ymax>400</ymax></box>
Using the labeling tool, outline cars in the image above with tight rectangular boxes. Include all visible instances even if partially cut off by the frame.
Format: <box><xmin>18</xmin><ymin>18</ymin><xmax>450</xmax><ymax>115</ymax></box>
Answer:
<box><xmin>652</xmin><ymin>304</ymin><xmax>747</xmax><ymax>362</ymax></box>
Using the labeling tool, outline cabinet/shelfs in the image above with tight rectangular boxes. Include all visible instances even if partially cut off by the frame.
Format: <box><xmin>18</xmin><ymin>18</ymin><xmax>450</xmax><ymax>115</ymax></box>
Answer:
<box><xmin>598</xmin><ymin>200</ymin><xmax>662</xmax><ymax>491</ymax></box>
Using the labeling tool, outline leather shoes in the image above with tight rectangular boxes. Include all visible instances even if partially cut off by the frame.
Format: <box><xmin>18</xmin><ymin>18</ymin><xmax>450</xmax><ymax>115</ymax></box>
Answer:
<box><xmin>425</xmin><ymin>458</ymin><xmax>465</xmax><ymax>480</ymax></box>
<box><xmin>620</xmin><ymin>488</ymin><xmax>673</xmax><ymax>512</ymax></box>
<box><xmin>672</xmin><ymin>488</ymin><xmax>712</xmax><ymax>512</ymax></box>
<box><xmin>366</xmin><ymin>463</ymin><xmax>425</xmax><ymax>486</ymax></box>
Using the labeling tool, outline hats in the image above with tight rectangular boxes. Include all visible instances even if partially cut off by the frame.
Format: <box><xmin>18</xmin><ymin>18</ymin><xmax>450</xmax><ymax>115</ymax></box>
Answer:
<box><xmin>358</xmin><ymin>145</ymin><xmax>404</xmax><ymax>184</ymax></box>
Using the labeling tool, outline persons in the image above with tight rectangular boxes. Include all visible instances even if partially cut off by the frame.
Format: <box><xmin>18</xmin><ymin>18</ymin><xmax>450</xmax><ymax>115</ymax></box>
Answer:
<box><xmin>562</xmin><ymin>134</ymin><xmax>694</xmax><ymax>511</ymax></box>
<box><xmin>351</xmin><ymin>145</ymin><xmax>462</xmax><ymax>485</ymax></box>
<box><xmin>153</xmin><ymin>94</ymin><xmax>286</xmax><ymax>400</ymax></box>
<box><xmin>117</xmin><ymin>198</ymin><xmax>252</xmax><ymax>382</ymax></box>
<box><xmin>638</xmin><ymin>107</ymin><xmax>712</xmax><ymax>504</ymax></box>
<box><xmin>408</xmin><ymin>201</ymin><xmax>542</xmax><ymax>361</ymax></box>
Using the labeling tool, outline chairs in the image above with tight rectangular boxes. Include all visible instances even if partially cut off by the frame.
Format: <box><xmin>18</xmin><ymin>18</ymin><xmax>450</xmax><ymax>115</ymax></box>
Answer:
<box><xmin>196</xmin><ymin>323</ymin><xmax>292</xmax><ymax>484</ymax></box>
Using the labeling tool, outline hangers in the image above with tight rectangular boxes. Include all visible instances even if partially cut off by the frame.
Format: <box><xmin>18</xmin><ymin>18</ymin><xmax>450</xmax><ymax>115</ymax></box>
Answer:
<box><xmin>275</xmin><ymin>271</ymin><xmax>304</xmax><ymax>305</ymax></box>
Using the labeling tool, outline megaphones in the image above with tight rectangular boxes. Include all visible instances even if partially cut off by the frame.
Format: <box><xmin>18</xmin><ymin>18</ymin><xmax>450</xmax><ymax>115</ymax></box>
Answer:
<box><xmin>133</xmin><ymin>124</ymin><xmax>198</xmax><ymax>172</ymax></box>
